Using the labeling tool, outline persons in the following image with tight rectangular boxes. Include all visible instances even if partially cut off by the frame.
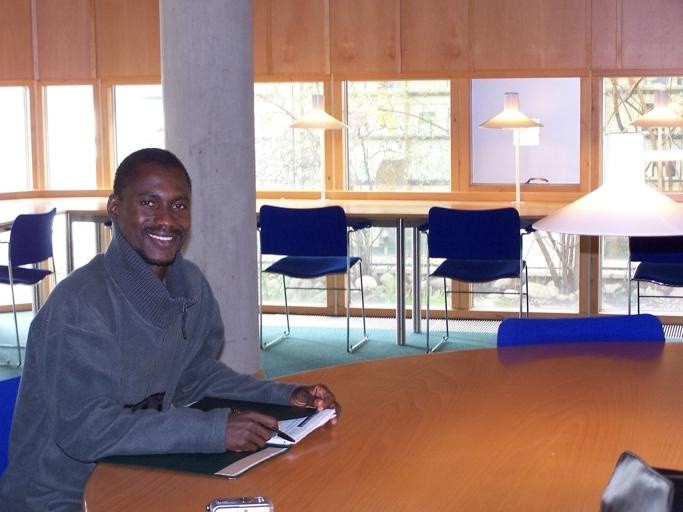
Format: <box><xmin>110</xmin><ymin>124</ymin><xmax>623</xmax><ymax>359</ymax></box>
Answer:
<box><xmin>0</xmin><ymin>148</ymin><xmax>342</xmax><ymax>512</ymax></box>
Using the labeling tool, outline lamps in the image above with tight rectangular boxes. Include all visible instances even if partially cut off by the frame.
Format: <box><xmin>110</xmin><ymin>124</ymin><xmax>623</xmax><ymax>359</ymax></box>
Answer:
<box><xmin>478</xmin><ymin>92</ymin><xmax>545</xmax><ymax>204</ymax></box>
<box><xmin>289</xmin><ymin>106</ymin><xmax>350</xmax><ymax>201</ymax></box>
<box><xmin>629</xmin><ymin>91</ymin><xmax>683</xmax><ymax>192</ymax></box>
<box><xmin>532</xmin><ymin>133</ymin><xmax>683</xmax><ymax>238</ymax></box>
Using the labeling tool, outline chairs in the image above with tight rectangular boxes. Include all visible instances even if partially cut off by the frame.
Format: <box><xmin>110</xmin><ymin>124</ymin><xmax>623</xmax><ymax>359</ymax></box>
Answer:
<box><xmin>629</xmin><ymin>236</ymin><xmax>683</xmax><ymax>314</ymax></box>
<box><xmin>260</xmin><ymin>205</ymin><xmax>369</xmax><ymax>353</ymax></box>
<box><xmin>426</xmin><ymin>207</ymin><xmax>529</xmax><ymax>354</ymax></box>
<box><xmin>496</xmin><ymin>314</ymin><xmax>665</xmax><ymax>348</ymax></box>
<box><xmin>1</xmin><ymin>208</ymin><xmax>57</xmax><ymax>366</ymax></box>
<box><xmin>0</xmin><ymin>376</ymin><xmax>21</xmax><ymax>475</ymax></box>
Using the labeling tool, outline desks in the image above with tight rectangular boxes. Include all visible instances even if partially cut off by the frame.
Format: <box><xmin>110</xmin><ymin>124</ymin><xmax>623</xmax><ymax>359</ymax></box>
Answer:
<box><xmin>84</xmin><ymin>343</ymin><xmax>683</xmax><ymax>512</ymax></box>
<box><xmin>0</xmin><ymin>197</ymin><xmax>574</xmax><ymax>345</ymax></box>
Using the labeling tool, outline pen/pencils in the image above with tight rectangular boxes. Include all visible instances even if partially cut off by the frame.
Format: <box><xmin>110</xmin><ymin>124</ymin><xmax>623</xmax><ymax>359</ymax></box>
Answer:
<box><xmin>238</xmin><ymin>411</ymin><xmax>295</xmax><ymax>443</ymax></box>
